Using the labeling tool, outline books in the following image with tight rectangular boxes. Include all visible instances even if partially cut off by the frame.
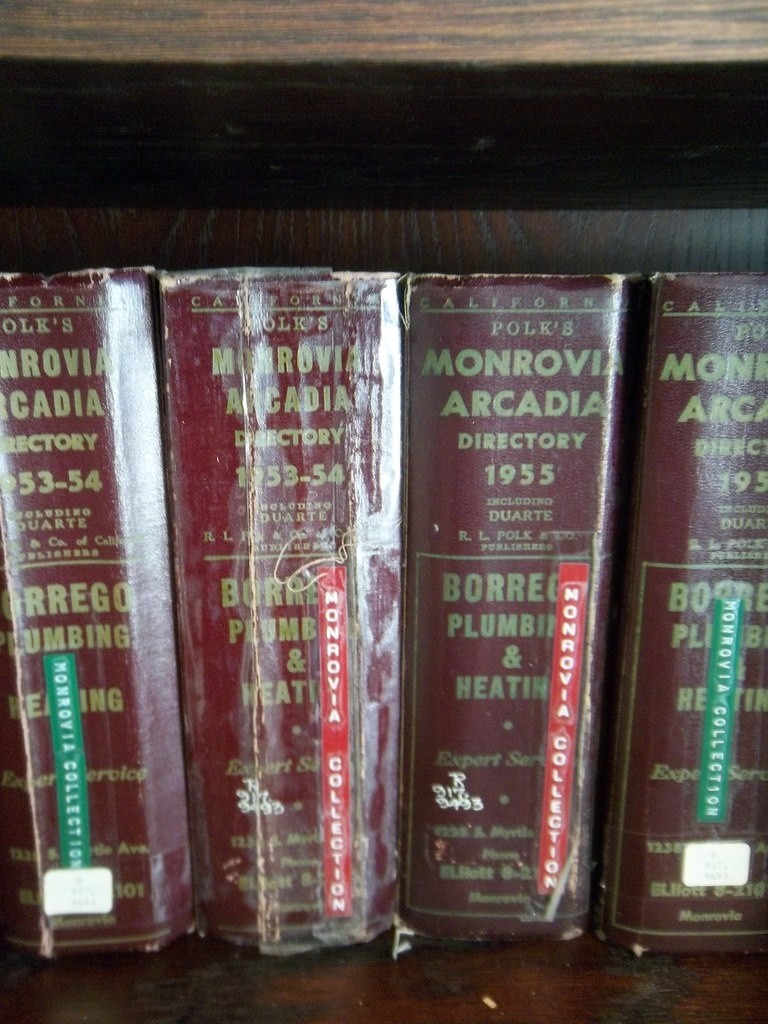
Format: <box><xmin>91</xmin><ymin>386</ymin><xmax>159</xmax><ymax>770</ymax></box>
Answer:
<box><xmin>0</xmin><ymin>267</ymin><xmax>768</xmax><ymax>960</ymax></box>
